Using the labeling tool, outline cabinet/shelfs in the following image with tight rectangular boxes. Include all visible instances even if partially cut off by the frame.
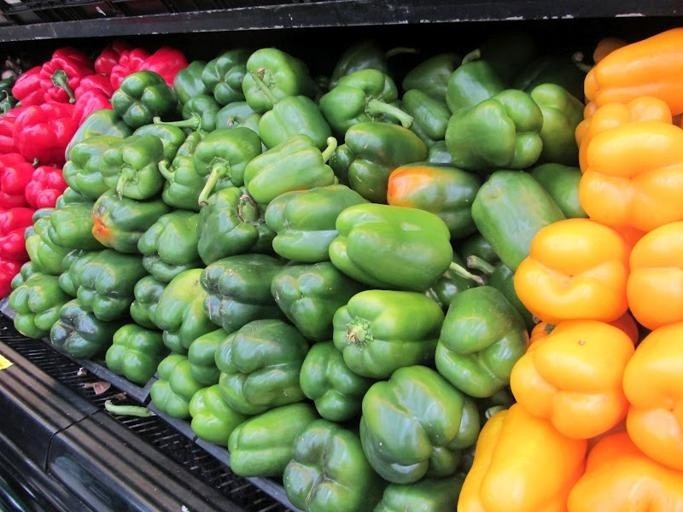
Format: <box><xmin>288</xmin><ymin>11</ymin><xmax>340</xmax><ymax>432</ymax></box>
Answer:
<box><xmin>0</xmin><ymin>1</ymin><xmax>680</xmax><ymax>511</ymax></box>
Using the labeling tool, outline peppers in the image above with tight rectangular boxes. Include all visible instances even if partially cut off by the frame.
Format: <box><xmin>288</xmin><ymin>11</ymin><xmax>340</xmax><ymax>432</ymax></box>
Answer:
<box><xmin>0</xmin><ymin>27</ymin><xmax>683</xmax><ymax>512</ymax></box>
